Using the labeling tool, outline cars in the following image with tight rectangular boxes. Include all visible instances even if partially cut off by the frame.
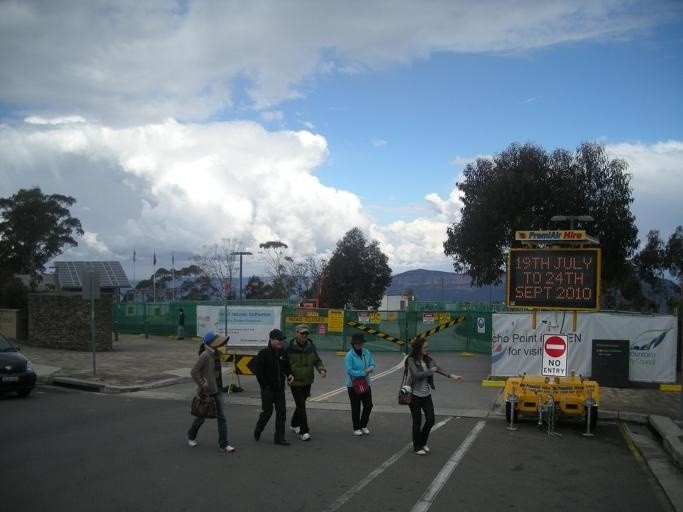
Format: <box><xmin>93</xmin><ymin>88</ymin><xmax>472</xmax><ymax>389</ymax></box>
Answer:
<box><xmin>0</xmin><ymin>333</ymin><xmax>37</xmax><ymax>398</ymax></box>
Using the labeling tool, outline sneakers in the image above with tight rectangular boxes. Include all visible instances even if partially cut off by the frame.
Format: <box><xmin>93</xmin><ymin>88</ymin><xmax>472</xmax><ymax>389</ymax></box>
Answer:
<box><xmin>353</xmin><ymin>430</ymin><xmax>363</xmax><ymax>436</ymax></box>
<box><xmin>274</xmin><ymin>438</ymin><xmax>290</xmax><ymax>445</ymax></box>
<box><xmin>254</xmin><ymin>431</ymin><xmax>261</xmax><ymax>441</ymax></box>
<box><xmin>186</xmin><ymin>435</ymin><xmax>198</xmax><ymax>447</ymax></box>
<box><xmin>416</xmin><ymin>446</ymin><xmax>429</xmax><ymax>455</ymax></box>
<box><xmin>219</xmin><ymin>445</ymin><xmax>235</xmax><ymax>452</ymax></box>
<box><xmin>299</xmin><ymin>432</ymin><xmax>310</xmax><ymax>441</ymax></box>
<box><xmin>361</xmin><ymin>428</ymin><xmax>370</xmax><ymax>435</ymax></box>
<box><xmin>290</xmin><ymin>425</ymin><xmax>301</xmax><ymax>434</ymax></box>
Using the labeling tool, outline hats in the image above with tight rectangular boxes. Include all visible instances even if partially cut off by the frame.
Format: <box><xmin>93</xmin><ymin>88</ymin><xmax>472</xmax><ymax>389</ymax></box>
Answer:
<box><xmin>295</xmin><ymin>324</ymin><xmax>309</xmax><ymax>334</ymax></box>
<box><xmin>203</xmin><ymin>331</ymin><xmax>230</xmax><ymax>348</ymax></box>
<box><xmin>350</xmin><ymin>333</ymin><xmax>368</xmax><ymax>345</ymax></box>
<box><xmin>268</xmin><ymin>329</ymin><xmax>287</xmax><ymax>341</ymax></box>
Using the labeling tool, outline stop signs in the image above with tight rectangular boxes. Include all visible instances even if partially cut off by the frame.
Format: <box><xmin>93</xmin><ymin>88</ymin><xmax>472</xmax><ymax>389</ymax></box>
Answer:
<box><xmin>544</xmin><ymin>336</ymin><xmax>565</xmax><ymax>358</ymax></box>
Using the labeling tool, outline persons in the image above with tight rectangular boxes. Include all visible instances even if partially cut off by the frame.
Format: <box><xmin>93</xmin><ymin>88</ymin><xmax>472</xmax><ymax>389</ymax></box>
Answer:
<box><xmin>404</xmin><ymin>337</ymin><xmax>464</xmax><ymax>455</ymax></box>
<box><xmin>253</xmin><ymin>328</ymin><xmax>295</xmax><ymax>446</ymax></box>
<box><xmin>176</xmin><ymin>307</ymin><xmax>186</xmax><ymax>340</ymax></box>
<box><xmin>344</xmin><ymin>331</ymin><xmax>377</xmax><ymax>436</ymax></box>
<box><xmin>185</xmin><ymin>331</ymin><xmax>236</xmax><ymax>452</ymax></box>
<box><xmin>285</xmin><ymin>323</ymin><xmax>328</xmax><ymax>441</ymax></box>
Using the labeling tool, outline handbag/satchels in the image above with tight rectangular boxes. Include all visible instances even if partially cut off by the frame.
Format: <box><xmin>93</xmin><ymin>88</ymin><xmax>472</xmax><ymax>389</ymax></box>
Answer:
<box><xmin>398</xmin><ymin>385</ymin><xmax>413</xmax><ymax>405</ymax></box>
<box><xmin>352</xmin><ymin>377</ymin><xmax>369</xmax><ymax>395</ymax></box>
<box><xmin>191</xmin><ymin>394</ymin><xmax>220</xmax><ymax>418</ymax></box>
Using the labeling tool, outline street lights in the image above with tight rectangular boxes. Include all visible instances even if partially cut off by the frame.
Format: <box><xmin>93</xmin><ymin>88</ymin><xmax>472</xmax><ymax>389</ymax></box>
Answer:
<box><xmin>550</xmin><ymin>214</ymin><xmax>594</xmax><ymax>232</ymax></box>
<box><xmin>230</xmin><ymin>251</ymin><xmax>252</xmax><ymax>304</ymax></box>
